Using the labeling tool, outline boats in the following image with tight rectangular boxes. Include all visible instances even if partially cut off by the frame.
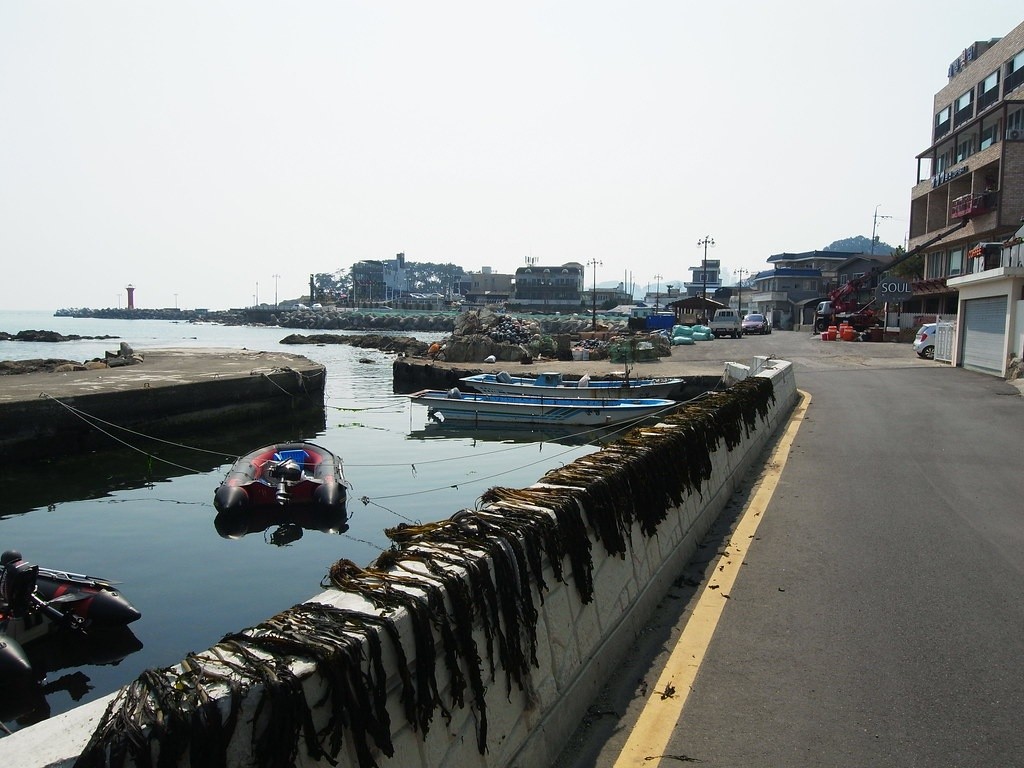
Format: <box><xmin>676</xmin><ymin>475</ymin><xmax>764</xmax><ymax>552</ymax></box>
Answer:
<box><xmin>407</xmin><ymin>388</ymin><xmax>675</xmax><ymax>426</ymax></box>
<box><xmin>0</xmin><ymin>547</ymin><xmax>140</xmax><ymax>675</ymax></box>
<box><xmin>213</xmin><ymin>441</ymin><xmax>347</xmax><ymax>519</ymax></box>
<box><xmin>458</xmin><ymin>370</ymin><xmax>685</xmax><ymax>401</ymax></box>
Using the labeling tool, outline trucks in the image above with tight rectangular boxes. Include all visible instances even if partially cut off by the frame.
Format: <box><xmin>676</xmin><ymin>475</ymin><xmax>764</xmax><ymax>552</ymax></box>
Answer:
<box><xmin>708</xmin><ymin>308</ymin><xmax>744</xmax><ymax>339</ymax></box>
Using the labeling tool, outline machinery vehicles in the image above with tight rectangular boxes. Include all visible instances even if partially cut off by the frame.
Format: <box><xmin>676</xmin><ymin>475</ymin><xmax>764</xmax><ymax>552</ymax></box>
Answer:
<box><xmin>813</xmin><ymin>218</ymin><xmax>968</xmax><ymax>332</ymax></box>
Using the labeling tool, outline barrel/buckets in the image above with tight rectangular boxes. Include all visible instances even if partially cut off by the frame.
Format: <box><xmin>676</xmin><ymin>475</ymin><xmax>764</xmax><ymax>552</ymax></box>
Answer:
<box><xmin>822</xmin><ymin>323</ymin><xmax>854</xmax><ymax>341</ymax></box>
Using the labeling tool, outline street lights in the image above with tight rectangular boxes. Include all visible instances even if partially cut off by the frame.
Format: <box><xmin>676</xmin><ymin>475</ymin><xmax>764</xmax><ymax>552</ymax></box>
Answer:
<box><xmin>653</xmin><ymin>273</ymin><xmax>663</xmax><ymax>316</ymax></box>
<box><xmin>697</xmin><ymin>235</ymin><xmax>715</xmax><ymax>326</ymax></box>
<box><xmin>586</xmin><ymin>258</ymin><xmax>602</xmax><ymax>332</ymax></box>
<box><xmin>732</xmin><ymin>268</ymin><xmax>748</xmax><ymax>313</ymax></box>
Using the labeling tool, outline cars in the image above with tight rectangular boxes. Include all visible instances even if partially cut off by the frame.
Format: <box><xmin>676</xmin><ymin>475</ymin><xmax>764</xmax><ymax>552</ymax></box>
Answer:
<box><xmin>912</xmin><ymin>323</ymin><xmax>936</xmax><ymax>359</ymax></box>
<box><xmin>741</xmin><ymin>314</ymin><xmax>767</xmax><ymax>335</ymax></box>
